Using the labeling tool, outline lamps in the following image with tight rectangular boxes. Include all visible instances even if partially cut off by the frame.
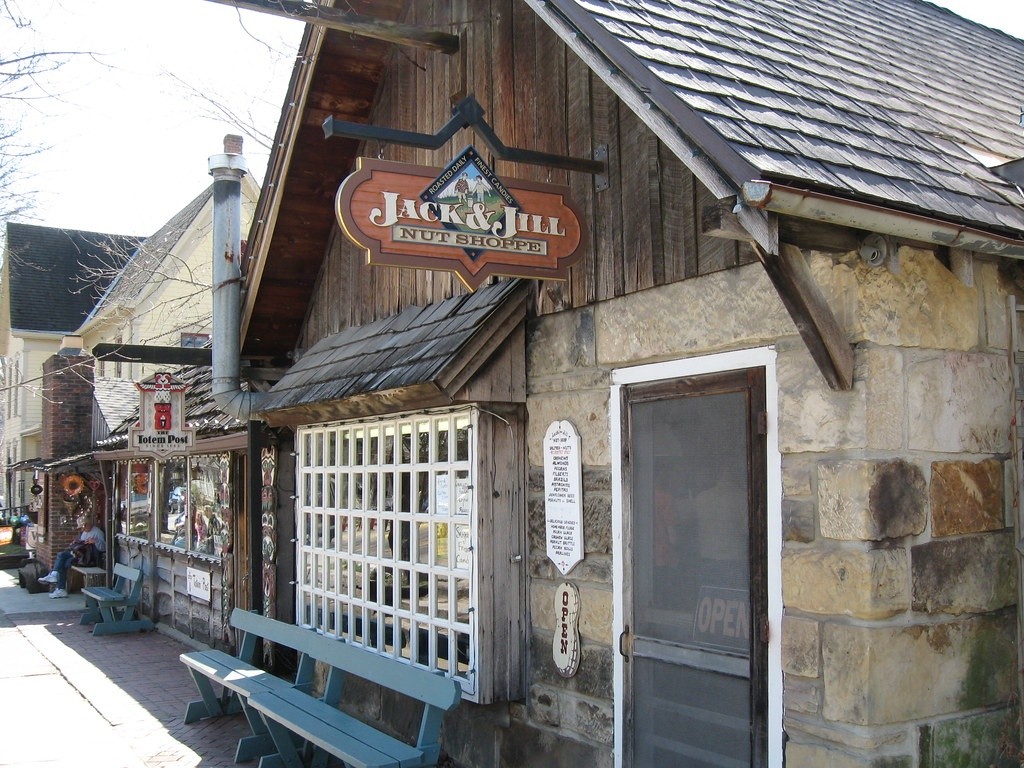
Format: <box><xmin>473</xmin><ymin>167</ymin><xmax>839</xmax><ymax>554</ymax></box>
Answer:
<box><xmin>860</xmin><ymin>235</ymin><xmax>888</xmax><ymax>267</ymax></box>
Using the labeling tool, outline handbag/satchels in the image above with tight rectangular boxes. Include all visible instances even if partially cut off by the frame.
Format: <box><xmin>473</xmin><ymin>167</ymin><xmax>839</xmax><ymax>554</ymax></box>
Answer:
<box><xmin>71</xmin><ymin>539</ymin><xmax>98</xmax><ymax>567</ymax></box>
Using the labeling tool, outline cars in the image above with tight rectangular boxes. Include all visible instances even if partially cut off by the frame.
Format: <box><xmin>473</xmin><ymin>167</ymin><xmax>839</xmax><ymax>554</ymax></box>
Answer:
<box><xmin>170</xmin><ymin>486</ymin><xmax>186</xmax><ymax>514</ymax></box>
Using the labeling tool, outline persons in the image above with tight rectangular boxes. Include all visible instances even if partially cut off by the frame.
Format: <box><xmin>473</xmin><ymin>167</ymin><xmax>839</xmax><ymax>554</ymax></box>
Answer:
<box><xmin>37</xmin><ymin>515</ymin><xmax>107</xmax><ymax>597</ymax></box>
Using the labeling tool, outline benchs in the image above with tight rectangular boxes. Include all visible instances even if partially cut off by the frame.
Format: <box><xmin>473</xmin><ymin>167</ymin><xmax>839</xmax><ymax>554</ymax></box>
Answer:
<box><xmin>180</xmin><ymin>606</ymin><xmax>463</xmax><ymax>767</ymax></box>
<box><xmin>69</xmin><ymin>565</ymin><xmax>106</xmax><ymax>607</ymax></box>
<box><xmin>81</xmin><ymin>563</ymin><xmax>154</xmax><ymax>636</ymax></box>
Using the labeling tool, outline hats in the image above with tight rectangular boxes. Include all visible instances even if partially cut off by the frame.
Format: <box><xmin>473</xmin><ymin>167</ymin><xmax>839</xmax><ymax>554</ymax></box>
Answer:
<box><xmin>76</xmin><ymin>516</ymin><xmax>89</xmax><ymax>529</ymax></box>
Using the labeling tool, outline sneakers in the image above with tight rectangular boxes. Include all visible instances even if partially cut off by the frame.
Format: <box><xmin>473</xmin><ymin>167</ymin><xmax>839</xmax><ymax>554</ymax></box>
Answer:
<box><xmin>38</xmin><ymin>573</ymin><xmax>58</xmax><ymax>585</ymax></box>
<box><xmin>48</xmin><ymin>588</ymin><xmax>68</xmax><ymax>598</ymax></box>
